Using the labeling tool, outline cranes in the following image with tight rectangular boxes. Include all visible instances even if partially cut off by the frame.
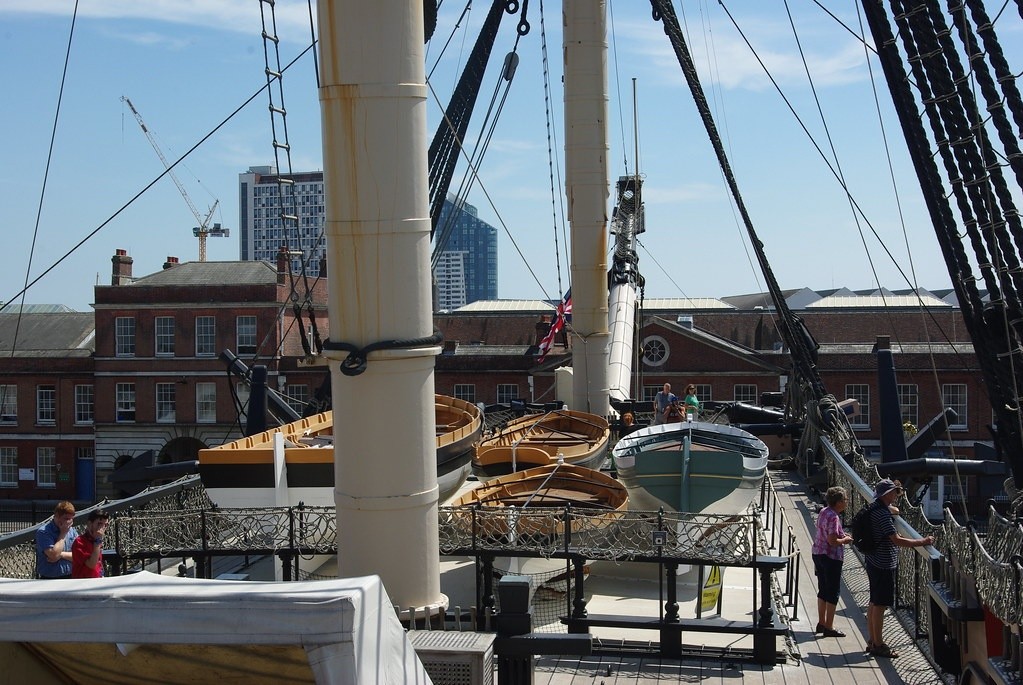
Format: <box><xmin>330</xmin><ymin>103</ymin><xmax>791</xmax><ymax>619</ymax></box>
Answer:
<box><xmin>119</xmin><ymin>96</ymin><xmax>230</xmax><ymax>262</ymax></box>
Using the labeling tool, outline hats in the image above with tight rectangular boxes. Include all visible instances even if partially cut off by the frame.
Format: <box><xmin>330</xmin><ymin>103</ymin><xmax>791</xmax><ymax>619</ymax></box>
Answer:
<box><xmin>872</xmin><ymin>479</ymin><xmax>901</xmax><ymax>499</ymax></box>
<box><xmin>671</xmin><ymin>397</ymin><xmax>679</xmax><ymax>401</ymax></box>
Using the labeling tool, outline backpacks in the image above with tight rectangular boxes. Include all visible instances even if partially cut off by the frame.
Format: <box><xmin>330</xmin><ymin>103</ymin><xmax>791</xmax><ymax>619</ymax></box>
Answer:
<box><xmin>851</xmin><ymin>499</ymin><xmax>882</xmax><ymax>557</ymax></box>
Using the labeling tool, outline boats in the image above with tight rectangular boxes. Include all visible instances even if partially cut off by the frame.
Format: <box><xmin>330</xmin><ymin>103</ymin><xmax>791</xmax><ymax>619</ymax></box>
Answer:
<box><xmin>449</xmin><ymin>452</ymin><xmax>629</xmax><ymax>606</ymax></box>
<box><xmin>197</xmin><ymin>393</ymin><xmax>484</xmax><ymax>581</ymax></box>
<box><xmin>470</xmin><ymin>404</ymin><xmax>611</xmax><ymax>484</ymax></box>
<box><xmin>612</xmin><ymin>412</ymin><xmax>770</xmax><ymax>576</ymax></box>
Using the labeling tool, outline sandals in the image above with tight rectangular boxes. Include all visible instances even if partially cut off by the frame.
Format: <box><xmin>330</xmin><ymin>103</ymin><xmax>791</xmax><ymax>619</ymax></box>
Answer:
<box><xmin>870</xmin><ymin>642</ymin><xmax>898</xmax><ymax>658</ymax></box>
<box><xmin>866</xmin><ymin>640</ymin><xmax>874</xmax><ymax>653</ymax></box>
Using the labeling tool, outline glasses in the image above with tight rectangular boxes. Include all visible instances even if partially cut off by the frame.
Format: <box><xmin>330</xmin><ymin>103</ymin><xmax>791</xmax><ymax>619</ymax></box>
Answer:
<box><xmin>687</xmin><ymin>388</ymin><xmax>695</xmax><ymax>390</ymax></box>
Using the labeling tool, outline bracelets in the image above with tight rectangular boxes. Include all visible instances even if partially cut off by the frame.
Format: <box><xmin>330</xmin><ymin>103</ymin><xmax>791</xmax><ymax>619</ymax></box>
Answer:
<box><xmin>94</xmin><ymin>536</ymin><xmax>103</xmax><ymax>544</ymax></box>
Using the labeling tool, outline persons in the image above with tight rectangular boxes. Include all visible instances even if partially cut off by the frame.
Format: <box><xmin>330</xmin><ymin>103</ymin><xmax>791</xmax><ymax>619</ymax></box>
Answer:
<box><xmin>70</xmin><ymin>508</ymin><xmax>108</xmax><ymax>578</ymax></box>
<box><xmin>653</xmin><ymin>383</ymin><xmax>675</xmax><ymax>425</ymax></box>
<box><xmin>811</xmin><ymin>486</ymin><xmax>855</xmax><ymax>637</ymax></box>
<box><xmin>866</xmin><ymin>478</ymin><xmax>935</xmax><ymax>657</ymax></box>
<box><xmin>685</xmin><ymin>384</ymin><xmax>699</xmax><ymax>422</ymax></box>
<box><xmin>617</xmin><ymin>412</ymin><xmax>639</xmax><ymax>439</ymax></box>
<box><xmin>34</xmin><ymin>500</ymin><xmax>79</xmax><ymax>578</ymax></box>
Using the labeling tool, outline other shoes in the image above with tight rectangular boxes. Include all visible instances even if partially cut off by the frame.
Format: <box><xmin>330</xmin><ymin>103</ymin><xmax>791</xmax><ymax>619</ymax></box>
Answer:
<box><xmin>823</xmin><ymin>627</ymin><xmax>846</xmax><ymax>637</ymax></box>
<box><xmin>816</xmin><ymin>622</ymin><xmax>826</xmax><ymax>633</ymax></box>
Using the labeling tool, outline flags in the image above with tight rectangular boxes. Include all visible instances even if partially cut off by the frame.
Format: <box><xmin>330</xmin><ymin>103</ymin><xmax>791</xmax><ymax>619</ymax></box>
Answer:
<box><xmin>537</xmin><ymin>287</ymin><xmax>572</xmax><ymax>364</ymax></box>
